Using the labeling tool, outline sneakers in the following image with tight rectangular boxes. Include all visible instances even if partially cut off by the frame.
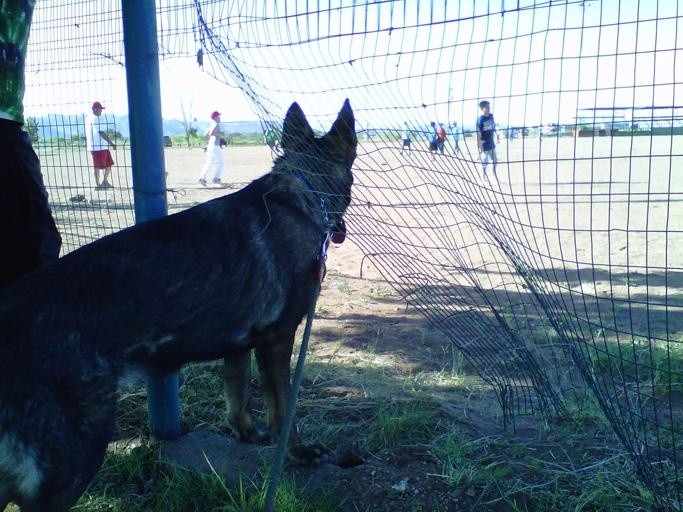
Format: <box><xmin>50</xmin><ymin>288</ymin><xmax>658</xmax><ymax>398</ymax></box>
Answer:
<box><xmin>199</xmin><ymin>177</ymin><xmax>225</xmax><ymax>187</ymax></box>
<box><xmin>93</xmin><ymin>180</ymin><xmax>114</xmax><ymax>192</ymax></box>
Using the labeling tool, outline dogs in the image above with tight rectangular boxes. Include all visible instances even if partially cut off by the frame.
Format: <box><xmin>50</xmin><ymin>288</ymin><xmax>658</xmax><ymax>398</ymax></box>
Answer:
<box><xmin>0</xmin><ymin>97</ymin><xmax>359</xmax><ymax>512</ymax></box>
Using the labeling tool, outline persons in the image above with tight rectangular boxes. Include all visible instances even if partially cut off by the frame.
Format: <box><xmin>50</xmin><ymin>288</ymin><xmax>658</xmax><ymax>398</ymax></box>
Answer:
<box><xmin>84</xmin><ymin>101</ymin><xmax>117</xmax><ymax>191</ymax></box>
<box><xmin>264</xmin><ymin>125</ymin><xmax>281</xmax><ymax>161</ymax></box>
<box><xmin>449</xmin><ymin>121</ymin><xmax>459</xmax><ymax>153</ymax></box>
<box><xmin>198</xmin><ymin>111</ymin><xmax>224</xmax><ymax>186</ymax></box>
<box><xmin>438</xmin><ymin>122</ymin><xmax>446</xmax><ymax>154</ymax></box>
<box><xmin>0</xmin><ymin>0</ymin><xmax>64</xmax><ymax>277</ymax></box>
<box><xmin>401</xmin><ymin>121</ymin><xmax>414</xmax><ymax>156</ymax></box>
<box><xmin>429</xmin><ymin>120</ymin><xmax>437</xmax><ymax>161</ymax></box>
<box><xmin>475</xmin><ymin>99</ymin><xmax>504</xmax><ymax>182</ymax></box>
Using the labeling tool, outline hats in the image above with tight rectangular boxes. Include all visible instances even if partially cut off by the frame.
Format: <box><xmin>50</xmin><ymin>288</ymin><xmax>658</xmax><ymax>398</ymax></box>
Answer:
<box><xmin>209</xmin><ymin>111</ymin><xmax>222</xmax><ymax>119</ymax></box>
<box><xmin>90</xmin><ymin>102</ymin><xmax>105</xmax><ymax>110</ymax></box>
